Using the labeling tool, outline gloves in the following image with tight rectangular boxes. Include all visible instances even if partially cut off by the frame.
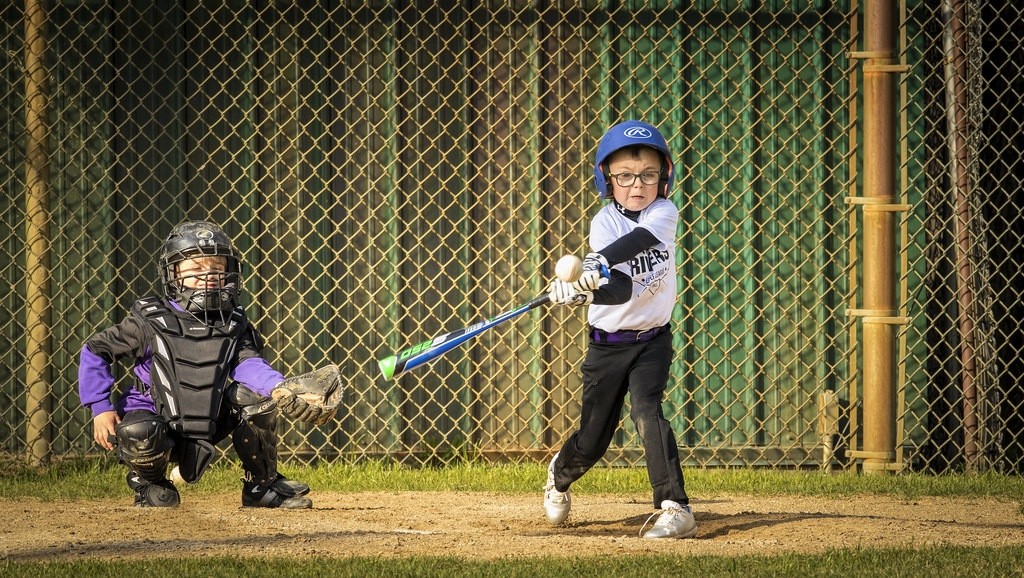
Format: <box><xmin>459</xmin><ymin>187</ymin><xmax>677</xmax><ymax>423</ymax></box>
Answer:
<box><xmin>571</xmin><ymin>253</ymin><xmax>609</xmax><ymax>291</ymax></box>
<box><xmin>547</xmin><ymin>278</ymin><xmax>594</xmax><ymax>306</ymax></box>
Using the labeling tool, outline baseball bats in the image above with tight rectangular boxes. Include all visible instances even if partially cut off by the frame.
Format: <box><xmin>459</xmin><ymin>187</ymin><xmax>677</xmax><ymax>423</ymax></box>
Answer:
<box><xmin>376</xmin><ymin>265</ymin><xmax>611</xmax><ymax>383</ymax></box>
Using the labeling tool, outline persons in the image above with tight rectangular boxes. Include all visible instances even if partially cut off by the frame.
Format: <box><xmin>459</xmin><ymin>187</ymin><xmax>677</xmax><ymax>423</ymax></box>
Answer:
<box><xmin>542</xmin><ymin>119</ymin><xmax>698</xmax><ymax>540</ymax></box>
<box><xmin>78</xmin><ymin>220</ymin><xmax>345</xmax><ymax>510</ymax></box>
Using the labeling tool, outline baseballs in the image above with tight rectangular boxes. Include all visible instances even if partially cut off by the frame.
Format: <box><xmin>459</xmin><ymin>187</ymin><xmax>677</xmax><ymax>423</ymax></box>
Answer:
<box><xmin>554</xmin><ymin>253</ymin><xmax>585</xmax><ymax>283</ymax></box>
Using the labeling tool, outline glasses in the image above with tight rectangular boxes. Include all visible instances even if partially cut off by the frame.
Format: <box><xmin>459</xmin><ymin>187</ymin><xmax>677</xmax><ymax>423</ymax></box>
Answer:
<box><xmin>607</xmin><ymin>166</ymin><xmax>664</xmax><ymax>187</ymax></box>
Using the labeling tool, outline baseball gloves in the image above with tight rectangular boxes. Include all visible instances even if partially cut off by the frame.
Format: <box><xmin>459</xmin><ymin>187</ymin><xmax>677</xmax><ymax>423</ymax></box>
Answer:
<box><xmin>269</xmin><ymin>363</ymin><xmax>346</xmax><ymax>427</ymax></box>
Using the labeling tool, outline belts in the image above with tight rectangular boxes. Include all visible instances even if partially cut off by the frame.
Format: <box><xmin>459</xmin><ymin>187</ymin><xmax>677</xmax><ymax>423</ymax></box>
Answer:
<box><xmin>595</xmin><ymin>328</ymin><xmax>660</xmax><ymax>343</ymax></box>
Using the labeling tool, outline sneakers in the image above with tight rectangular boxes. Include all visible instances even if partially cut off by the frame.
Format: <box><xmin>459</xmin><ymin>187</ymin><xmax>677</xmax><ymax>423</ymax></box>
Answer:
<box><xmin>133</xmin><ymin>491</ymin><xmax>152</xmax><ymax>509</ymax></box>
<box><xmin>544</xmin><ymin>452</ymin><xmax>571</xmax><ymax>524</ymax></box>
<box><xmin>240</xmin><ymin>478</ymin><xmax>313</xmax><ymax>509</ymax></box>
<box><xmin>641</xmin><ymin>499</ymin><xmax>698</xmax><ymax>539</ymax></box>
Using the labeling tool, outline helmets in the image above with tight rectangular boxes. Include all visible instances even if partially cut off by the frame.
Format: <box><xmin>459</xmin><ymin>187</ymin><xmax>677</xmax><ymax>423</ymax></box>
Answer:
<box><xmin>159</xmin><ymin>220</ymin><xmax>244</xmax><ymax>328</ymax></box>
<box><xmin>593</xmin><ymin>120</ymin><xmax>675</xmax><ymax>200</ymax></box>
